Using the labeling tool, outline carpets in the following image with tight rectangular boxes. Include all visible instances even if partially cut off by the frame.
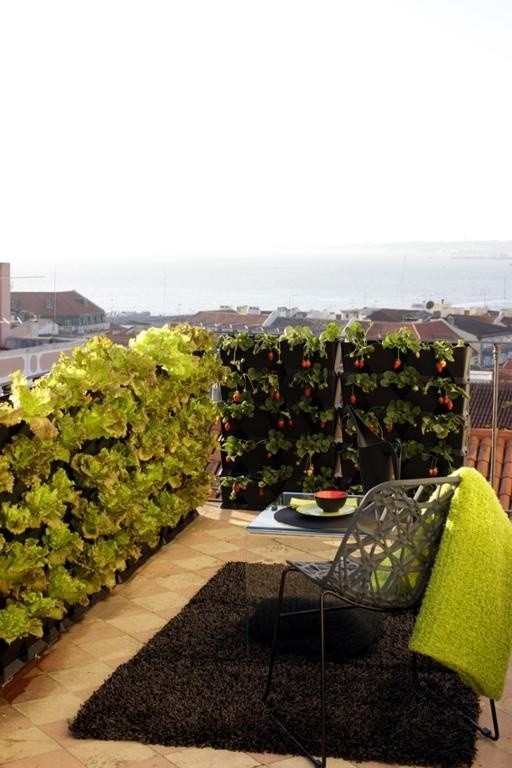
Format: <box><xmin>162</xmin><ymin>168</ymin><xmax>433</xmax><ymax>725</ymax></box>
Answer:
<box><xmin>70</xmin><ymin>562</ymin><xmax>480</xmax><ymax>766</ymax></box>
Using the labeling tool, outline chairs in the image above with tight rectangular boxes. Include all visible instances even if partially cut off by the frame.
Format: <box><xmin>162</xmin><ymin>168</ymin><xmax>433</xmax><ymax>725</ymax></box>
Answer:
<box><xmin>262</xmin><ymin>474</ymin><xmax>499</xmax><ymax>766</ymax></box>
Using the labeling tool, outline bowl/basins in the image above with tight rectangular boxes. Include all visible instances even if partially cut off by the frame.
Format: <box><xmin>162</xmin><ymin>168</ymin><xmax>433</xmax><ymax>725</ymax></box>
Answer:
<box><xmin>313</xmin><ymin>491</ymin><xmax>349</xmax><ymax>513</ymax></box>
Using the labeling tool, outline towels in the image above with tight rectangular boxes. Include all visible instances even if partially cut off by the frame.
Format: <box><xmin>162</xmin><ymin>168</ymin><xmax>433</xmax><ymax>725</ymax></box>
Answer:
<box><xmin>371</xmin><ymin>463</ymin><xmax>511</xmax><ymax>701</ymax></box>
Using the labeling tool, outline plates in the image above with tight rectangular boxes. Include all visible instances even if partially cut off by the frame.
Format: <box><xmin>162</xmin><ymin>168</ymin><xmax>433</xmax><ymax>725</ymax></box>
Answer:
<box><xmin>295</xmin><ymin>504</ymin><xmax>356</xmax><ymax>517</ymax></box>
